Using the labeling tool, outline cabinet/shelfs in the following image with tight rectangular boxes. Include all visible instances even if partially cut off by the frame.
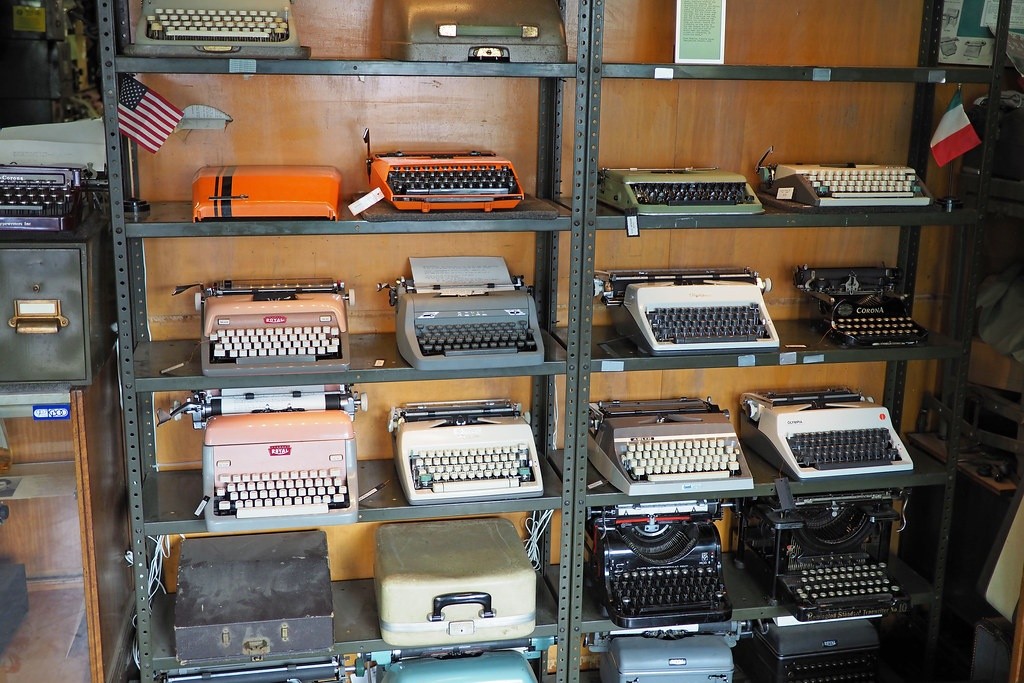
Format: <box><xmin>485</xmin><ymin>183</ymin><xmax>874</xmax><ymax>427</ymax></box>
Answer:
<box><xmin>94</xmin><ymin>1</ymin><xmax>589</xmax><ymax>683</ymax></box>
<box><xmin>0</xmin><ymin>220</ymin><xmax>138</xmax><ymax>683</ymax></box>
<box><xmin>547</xmin><ymin>0</ymin><xmax>1017</xmax><ymax>683</ymax></box>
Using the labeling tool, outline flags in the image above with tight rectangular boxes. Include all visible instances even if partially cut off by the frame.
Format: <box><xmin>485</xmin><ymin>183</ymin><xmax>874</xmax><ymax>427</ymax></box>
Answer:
<box><xmin>930</xmin><ymin>91</ymin><xmax>981</xmax><ymax>167</ymax></box>
<box><xmin>117</xmin><ymin>76</ymin><xmax>185</xmax><ymax>154</ymax></box>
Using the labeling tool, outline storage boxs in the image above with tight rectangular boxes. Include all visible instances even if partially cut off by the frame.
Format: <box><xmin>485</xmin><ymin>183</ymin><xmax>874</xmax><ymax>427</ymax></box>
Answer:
<box><xmin>598</xmin><ymin>632</ymin><xmax>736</xmax><ymax>683</ymax></box>
<box><xmin>174</xmin><ymin>531</ymin><xmax>334</xmax><ymax>662</ymax></box>
<box><xmin>373</xmin><ymin>515</ymin><xmax>536</xmax><ymax>647</ymax></box>
